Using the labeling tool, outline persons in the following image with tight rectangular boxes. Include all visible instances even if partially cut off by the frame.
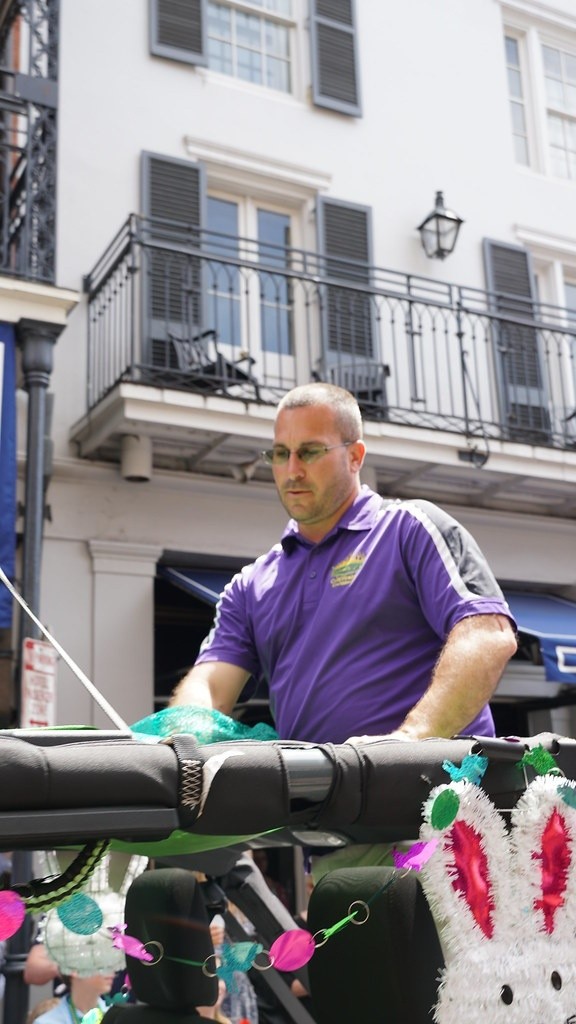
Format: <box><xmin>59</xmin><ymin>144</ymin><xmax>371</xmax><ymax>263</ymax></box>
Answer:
<box><xmin>0</xmin><ymin>856</ymin><xmax>316</xmax><ymax>1024</ymax></box>
<box><xmin>167</xmin><ymin>382</ymin><xmax>518</xmax><ymax>892</ymax></box>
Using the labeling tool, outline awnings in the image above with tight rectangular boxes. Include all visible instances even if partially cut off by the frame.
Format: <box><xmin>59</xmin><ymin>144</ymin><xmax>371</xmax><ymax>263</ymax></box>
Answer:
<box><xmin>504</xmin><ymin>591</ymin><xmax>576</xmax><ymax>684</ymax></box>
<box><xmin>160</xmin><ymin>565</ymin><xmax>240</xmax><ymax>609</ymax></box>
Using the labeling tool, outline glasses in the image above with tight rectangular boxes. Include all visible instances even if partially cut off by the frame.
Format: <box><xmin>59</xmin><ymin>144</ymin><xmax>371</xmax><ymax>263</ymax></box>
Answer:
<box><xmin>262</xmin><ymin>442</ymin><xmax>350</xmax><ymax>465</ymax></box>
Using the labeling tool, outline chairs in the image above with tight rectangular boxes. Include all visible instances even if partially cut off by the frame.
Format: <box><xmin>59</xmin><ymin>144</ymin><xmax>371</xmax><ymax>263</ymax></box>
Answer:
<box><xmin>312</xmin><ymin>360</ymin><xmax>391</xmax><ymax>419</ymax></box>
<box><xmin>148</xmin><ymin>328</ymin><xmax>262</xmax><ymax>402</ymax></box>
<box><xmin>100</xmin><ymin>871</ymin><xmax>227</xmax><ymax>1024</ymax></box>
<box><xmin>306</xmin><ymin>867</ymin><xmax>445</xmax><ymax>1024</ymax></box>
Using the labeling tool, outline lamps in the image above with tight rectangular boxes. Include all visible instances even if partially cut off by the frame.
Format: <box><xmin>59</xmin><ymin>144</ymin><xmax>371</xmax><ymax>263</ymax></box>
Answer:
<box><xmin>418</xmin><ymin>190</ymin><xmax>464</xmax><ymax>262</ymax></box>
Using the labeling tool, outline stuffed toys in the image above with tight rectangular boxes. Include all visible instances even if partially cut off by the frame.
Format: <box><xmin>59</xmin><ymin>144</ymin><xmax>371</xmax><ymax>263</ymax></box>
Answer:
<box><xmin>413</xmin><ymin>748</ymin><xmax>576</xmax><ymax>1024</ymax></box>
